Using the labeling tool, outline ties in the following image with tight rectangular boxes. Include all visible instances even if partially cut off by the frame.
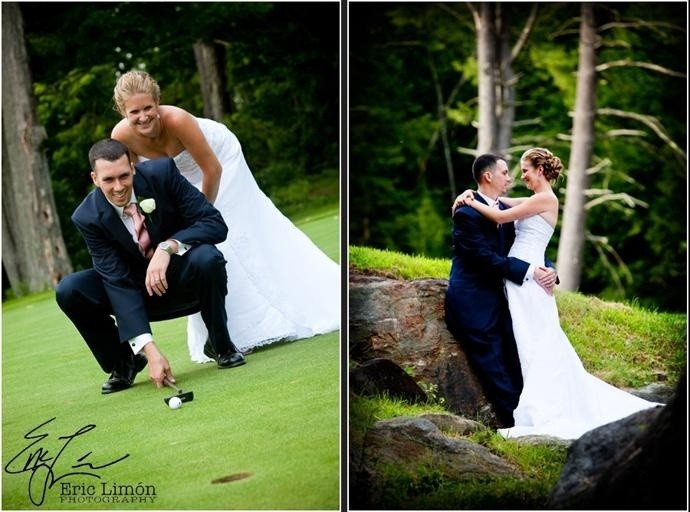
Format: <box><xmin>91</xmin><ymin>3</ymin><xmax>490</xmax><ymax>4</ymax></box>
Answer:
<box><xmin>124</xmin><ymin>204</ymin><xmax>154</xmax><ymax>261</ymax></box>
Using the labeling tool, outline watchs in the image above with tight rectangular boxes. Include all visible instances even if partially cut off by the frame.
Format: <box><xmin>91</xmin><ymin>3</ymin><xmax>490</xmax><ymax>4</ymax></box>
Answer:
<box><xmin>155</xmin><ymin>240</ymin><xmax>174</xmax><ymax>258</ymax></box>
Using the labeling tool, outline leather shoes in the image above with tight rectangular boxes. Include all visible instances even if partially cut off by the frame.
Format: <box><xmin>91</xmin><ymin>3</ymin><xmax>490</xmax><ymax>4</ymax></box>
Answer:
<box><xmin>204</xmin><ymin>339</ymin><xmax>247</xmax><ymax>368</ymax></box>
<box><xmin>101</xmin><ymin>352</ymin><xmax>147</xmax><ymax>395</ymax></box>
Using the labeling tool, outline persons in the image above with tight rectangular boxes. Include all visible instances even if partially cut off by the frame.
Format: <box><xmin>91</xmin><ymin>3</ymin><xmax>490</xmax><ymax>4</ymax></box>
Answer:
<box><xmin>110</xmin><ymin>70</ymin><xmax>340</xmax><ymax>363</ymax></box>
<box><xmin>445</xmin><ymin>151</ymin><xmax>558</xmax><ymax>428</ymax></box>
<box><xmin>56</xmin><ymin>138</ymin><xmax>248</xmax><ymax>395</ymax></box>
<box><xmin>450</xmin><ymin>146</ymin><xmax>667</xmax><ymax>440</ymax></box>
